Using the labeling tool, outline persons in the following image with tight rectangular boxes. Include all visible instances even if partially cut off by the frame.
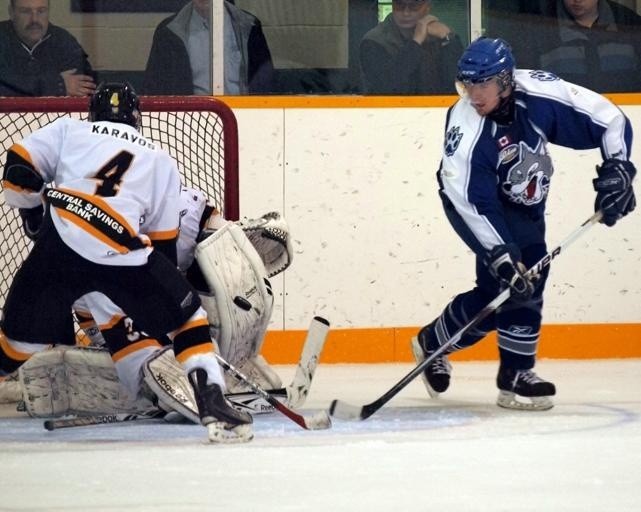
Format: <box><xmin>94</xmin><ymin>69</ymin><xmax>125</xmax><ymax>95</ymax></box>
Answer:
<box><xmin>347</xmin><ymin>0</ymin><xmax>466</xmax><ymax>95</ymax></box>
<box><xmin>0</xmin><ymin>72</ymin><xmax>254</xmax><ymax>445</ymax></box>
<box><xmin>536</xmin><ymin>0</ymin><xmax>641</xmax><ymax>94</ymax></box>
<box><xmin>412</xmin><ymin>35</ymin><xmax>638</xmax><ymax>411</ymax></box>
<box><xmin>71</xmin><ymin>183</ymin><xmax>295</xmax><ymax>422</ymax></box>
<box><xmin>139</xmin><ymin>0</ymin><xmax>284</xmax><ymax>95</ymax></box>
<box><xmin>0</xmin><ymin>0</ymin><xmax>98</xmax><ymax>96</ymax></box>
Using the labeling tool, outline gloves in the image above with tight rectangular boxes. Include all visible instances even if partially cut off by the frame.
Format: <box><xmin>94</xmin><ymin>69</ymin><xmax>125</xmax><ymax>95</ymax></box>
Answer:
<box><xmin>593</xmin><ymin>157</ymin><xmax>636</xmax><ymax>226</ymax></box>
<box><xmin>484</xmin><ymin>244</ymin><xmax>541</xmax><ymax>298</ymax></box>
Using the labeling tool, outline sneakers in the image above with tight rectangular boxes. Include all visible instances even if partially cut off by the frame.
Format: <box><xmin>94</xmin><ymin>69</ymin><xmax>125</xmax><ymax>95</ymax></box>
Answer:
<box><xmin>418</xmin><ymin>318</ymin><xmax>449</xmax><ymax>392</ymax></box>
<box><xmin>497</xmin><ymin>365</ymin><xmax>555</xmax><ymax>397</ymax></box>
<box><xmin>188</xmin><ymin>368</ymin><xmax>253</xmax><ymax>424</ymax></box>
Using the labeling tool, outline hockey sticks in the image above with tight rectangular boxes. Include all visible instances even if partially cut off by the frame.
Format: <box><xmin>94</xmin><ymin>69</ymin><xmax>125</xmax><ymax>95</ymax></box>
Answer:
<box><xmin>216</xmin><ymin>357</ymin><xmax>331</xmax><ymax>430</ymax></box>
<box><xmin>45</xmin><ymin>316</ymin><xmax>331</xmax><ymax>434</ymax></box>
<box><xmin>328</xmin><ymin>204</ymin><xmax>603</xmax><ymax>422</ymax></box>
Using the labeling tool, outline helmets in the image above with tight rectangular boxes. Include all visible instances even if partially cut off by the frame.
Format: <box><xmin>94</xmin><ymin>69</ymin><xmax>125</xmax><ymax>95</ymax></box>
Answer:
<box><xmin>455</xmin><ymin>36</ymin><xmax>516</xmax><ymax>103</ymax></box>
<box><xmin>88</xmin><ymin>81</ymin><xmax>142</xmax><ymax>132</ymax></box>
